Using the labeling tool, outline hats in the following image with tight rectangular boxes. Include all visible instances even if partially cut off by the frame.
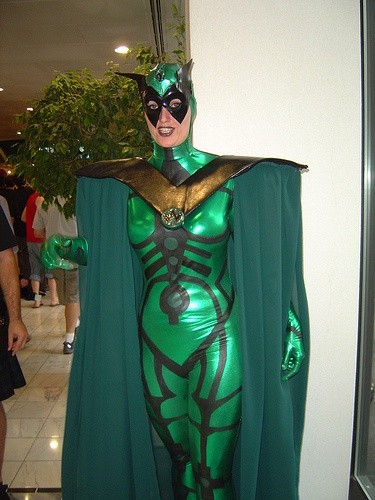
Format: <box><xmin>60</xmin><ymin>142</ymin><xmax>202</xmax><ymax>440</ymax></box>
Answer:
<box><xmin>115</xmin><ymin>58</ymin><xmax>196</xmax><ymax>98</ymax></box>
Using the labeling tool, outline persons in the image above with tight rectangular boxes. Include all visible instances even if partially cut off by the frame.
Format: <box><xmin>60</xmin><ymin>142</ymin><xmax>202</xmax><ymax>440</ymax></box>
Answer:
<box><xmin>38</xmin><ymin>58</ymin><xmax>310</xmax><ymax>500</ymax></box>
<box><xmin>21</xmin><ymin>188</ymin><xmax>61</xmax><ymax>309</ymax></box>
<box><xmin>32</xmin><ymin>192</ymin><xmax>81</xmax><ymax>354</ymax></box>
<box><xmin>0</xmin><ymin>204</ymin><xmax>31</xmax><ymax>500</ymax></box>
<box><xmin>0</xmin><ymin>168</ymin><xmax>35</xmax><ymax>300</ymax></box>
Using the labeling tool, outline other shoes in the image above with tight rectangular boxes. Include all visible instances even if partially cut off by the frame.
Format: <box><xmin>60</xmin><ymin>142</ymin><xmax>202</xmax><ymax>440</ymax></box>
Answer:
<box><xmin>62</xmin><ymin>338</ymin><xmax>74</xmax><ymax>354</ymax></box>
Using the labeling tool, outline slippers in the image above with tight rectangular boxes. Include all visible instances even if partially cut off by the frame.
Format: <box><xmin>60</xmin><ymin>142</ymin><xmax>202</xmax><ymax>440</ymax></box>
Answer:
<box><xmin>32</xmin><ymin>299</ymin><xmax>61</xmax><ymax>308</ymax></box>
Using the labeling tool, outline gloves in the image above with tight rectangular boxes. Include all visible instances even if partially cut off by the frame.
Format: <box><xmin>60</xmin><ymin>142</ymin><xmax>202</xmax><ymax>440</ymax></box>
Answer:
<box><xmin>281</xmin><ymin>322</ymin><xmax>306</xmax><ymax>381</ymax></box>
<box><xmin>41</xmin><ymin>233</ymin><xmax>77</xmax><ymax>270</ymax></box>
